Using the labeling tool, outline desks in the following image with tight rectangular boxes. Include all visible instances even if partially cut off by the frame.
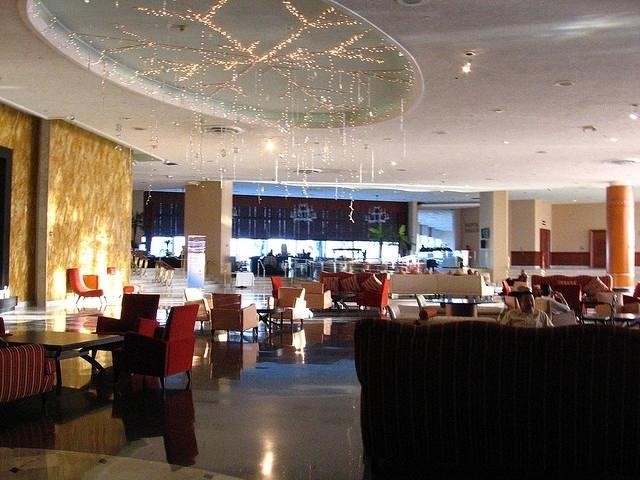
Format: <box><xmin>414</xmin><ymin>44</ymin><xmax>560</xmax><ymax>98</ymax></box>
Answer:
<box><xmin>2</xmin><ymin>330</ymin><xmax>124</xmax><ymax>422</ymax></box>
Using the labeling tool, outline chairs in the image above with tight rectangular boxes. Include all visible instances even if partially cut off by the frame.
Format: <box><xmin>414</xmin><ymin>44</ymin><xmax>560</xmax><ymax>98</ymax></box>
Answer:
<box><xmin>498</xmin><ymin>276</ymin><xmax>640</xmax><ymax>329</ymax></box>
<box><xmin>183</xmin><ymin>287</ymin><xmax>214</xmax><ymax>331</ymax></box>
<box><xmin>267</xmin><ymin>286</ymin><xmax>310</xmax><ymax>333</ymax></box>
<box><xmin>269</xmin><ymin>275</ymin><xmax>333</xmax><ymax>315</ymax></box>
<box><xmin>66</xmin><ymin>268</ymin><xmax>109</xmax><ymax>312</ymax></box>
<box><xmin>109</xmin><ymin>303</ymin><xmax>200</xmax><ymax>404</ymax></box>
<box><xmin>0</xmin><ymin>316</ymin><xmax>56</xmax><ymax>417</ymax></box>
<box><xmin>90</xmin><ymin>293</ymin><xmax>162</xmax><ymax>374</ymax></box>
<box><xmin>208</xmin><ymin>292</ymin><xmax>260</xmax><ymax>343</ymax></box>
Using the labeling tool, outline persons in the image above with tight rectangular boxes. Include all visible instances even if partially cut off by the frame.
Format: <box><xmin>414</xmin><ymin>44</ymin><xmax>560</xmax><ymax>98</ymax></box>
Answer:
<box><xmin>500</xmin><ymin>292</ymin><xmax>555</xmax><ymax>328</ymax></box>
<box><xmin>511</xmin><ymin>269</ymin><xmax>527</xmax><ymax>286</ymax></box>
<box><xmin>541</xmin><ymin>283</ymin><xmax>569</xmax><ymax>315</ymax></box>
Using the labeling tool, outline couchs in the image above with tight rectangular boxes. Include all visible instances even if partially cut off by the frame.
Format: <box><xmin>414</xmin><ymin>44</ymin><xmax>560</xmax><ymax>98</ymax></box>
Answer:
<box><xmin>353</xmin><ymin>316</ymin><xmax>640</xmax><ymax>478</ymax></box>
<box><xmin>321</xmin><ymin>268</ymin><xmax>496</xmax><ymax>314</ymax></box>
